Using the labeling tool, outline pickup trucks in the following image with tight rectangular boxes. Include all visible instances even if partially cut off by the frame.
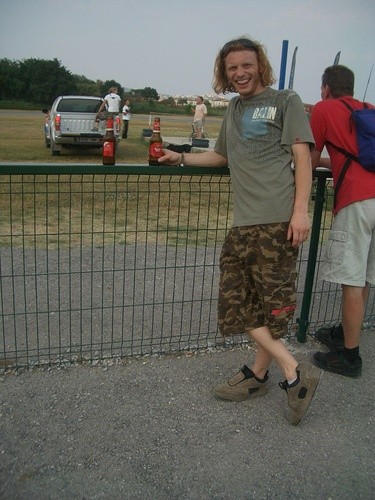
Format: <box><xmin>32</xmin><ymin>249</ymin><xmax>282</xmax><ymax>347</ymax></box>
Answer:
<box><xmin>42</xmin><ymin>95</ymin><xmax>122</xmax><ymax>157</ymax></box>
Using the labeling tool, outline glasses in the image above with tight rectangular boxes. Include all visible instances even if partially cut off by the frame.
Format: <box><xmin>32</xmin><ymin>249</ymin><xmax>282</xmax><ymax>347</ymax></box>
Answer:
<box><xmin>222</xmin><ymin>39</ymin><xmax>259</xmax><ymax>54</ymax></box>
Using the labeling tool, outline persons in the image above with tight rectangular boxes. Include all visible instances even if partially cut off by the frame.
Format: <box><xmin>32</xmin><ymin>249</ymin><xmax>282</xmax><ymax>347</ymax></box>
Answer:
<box><xmin>192</xmin><ymin>96</ymin><xmax>207</xmax><ymax>138</ymax></box>
<box><xmin>158</xmin><ymin>34</ymin><xmax>323</xmax><ymax>425</ymax></box>
<box><xmin>122</xmin><ymin>98</ymin><xmax>131</xmax><ymax>139</ymax></box>
<box><xmin>292</xmin><ymin>65</ymin><xmax>375</xmax><ymax>378</ymax></box>
<box><xmin>91</xmin><ymin>86</ymin><xmax>121</xmax><ymax>132</ymax></box>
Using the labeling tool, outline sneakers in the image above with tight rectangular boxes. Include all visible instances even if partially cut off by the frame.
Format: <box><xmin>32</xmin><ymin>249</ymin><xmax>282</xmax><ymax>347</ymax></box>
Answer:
<box><xmin>210</xmin><ymin>364</ymin><xmax>271</xmax><ymax>400</ymax></box>
<box><xmin>277</xmin><ymin>363</ymin><xmax>324</xmax><ymax>425</ymax></box>
<box><xmin>311</xmin><ymin>345</ymin><xmax>362</xmax><ymax>378</ymax></box>
<box><xmin>315</xmin><ymin>325</ymin><xmax>346</xmax><ymax>349</ymax></box>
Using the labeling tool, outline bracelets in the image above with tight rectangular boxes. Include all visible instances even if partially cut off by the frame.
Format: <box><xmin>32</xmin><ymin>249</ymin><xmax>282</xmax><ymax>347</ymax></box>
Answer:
<box><xmin>178</xmin><ymin>151</ymin><xmax>185</xmax><ymax>167</ymax></box>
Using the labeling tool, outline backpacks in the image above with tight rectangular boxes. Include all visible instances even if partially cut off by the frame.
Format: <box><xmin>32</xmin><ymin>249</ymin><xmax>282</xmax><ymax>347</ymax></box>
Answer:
<box><xmin>323</xmin><ymin>98</ymin><xmax>375</xmax><ymax>172</ymax></box>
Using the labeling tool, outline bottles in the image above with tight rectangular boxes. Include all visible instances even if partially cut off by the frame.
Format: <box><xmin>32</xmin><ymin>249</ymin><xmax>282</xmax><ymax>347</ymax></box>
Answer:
<box><xmin>101</xmin><ymin>116</ymin><xmax>117</xmax><ymax>166</ymax></box>
<box><xmin>148</xmin><ymin>116</ymin><xmax>163</xmax><ymax>166</ymax></box>
